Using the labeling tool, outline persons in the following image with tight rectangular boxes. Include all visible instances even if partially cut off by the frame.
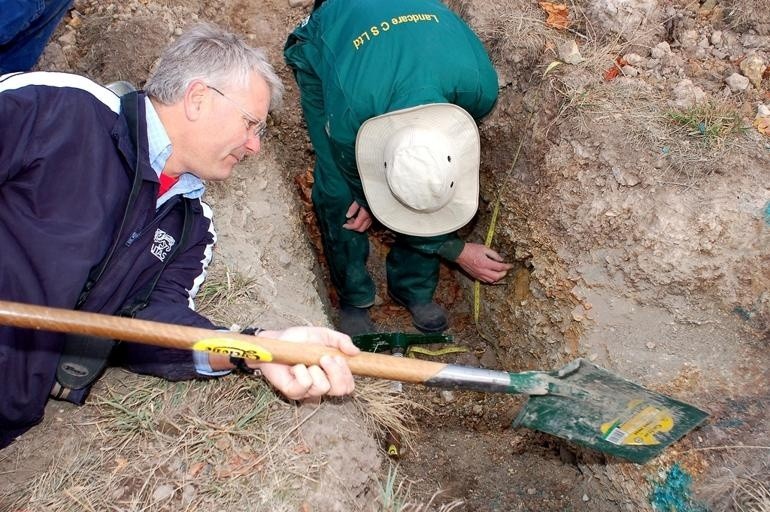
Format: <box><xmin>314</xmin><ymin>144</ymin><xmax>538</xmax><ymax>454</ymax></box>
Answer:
<box><xmin>0</xmin><ymin>22</ymin><xmax>363</xmax><ymax>449</ymax></box>
<box><xmin>282</xmin><ymin>0</ymin><xmax>516</xmax><ymax>334</ymax></box>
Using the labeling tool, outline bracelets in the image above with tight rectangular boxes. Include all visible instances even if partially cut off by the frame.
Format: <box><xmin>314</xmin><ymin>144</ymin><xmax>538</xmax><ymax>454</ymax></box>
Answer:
<box><xmin>229</xmin><ymin>328</ymin><xmax>265</xmax><ymax>377</ymax></box>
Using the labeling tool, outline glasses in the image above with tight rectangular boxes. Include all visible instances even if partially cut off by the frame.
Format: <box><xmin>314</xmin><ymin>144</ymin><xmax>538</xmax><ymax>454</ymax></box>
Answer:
<box><xmin>207</xmin><ymin>87</ymin><xmax>267</xmax><ymax>137</ymax></box>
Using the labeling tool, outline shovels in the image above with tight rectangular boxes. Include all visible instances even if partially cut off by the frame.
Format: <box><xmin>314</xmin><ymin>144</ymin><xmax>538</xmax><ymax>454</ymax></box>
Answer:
<box><xmin>1</xmin><ymin>298</ymin><xmax>712</xmax><ymax>467</ymax></box>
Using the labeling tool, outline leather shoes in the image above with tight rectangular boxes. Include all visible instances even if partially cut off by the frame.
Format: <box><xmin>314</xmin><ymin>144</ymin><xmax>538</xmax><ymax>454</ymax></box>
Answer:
<box><xmin>388</xmin><ymin>289</ymin><xmax>452</xmax><ymax>333</ymax></box>
<box><xmin>338</xmin><ymin>303</ymin><xmax>375</xmax><ymax>335</ymax></box>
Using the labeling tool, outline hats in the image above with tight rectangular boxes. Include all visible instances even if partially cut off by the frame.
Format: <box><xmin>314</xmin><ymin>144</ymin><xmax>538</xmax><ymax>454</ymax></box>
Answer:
<box><xmin>355</xmin><ymin>102</ymin><xmax>481</xmax><ymax>239</ymax></box>
